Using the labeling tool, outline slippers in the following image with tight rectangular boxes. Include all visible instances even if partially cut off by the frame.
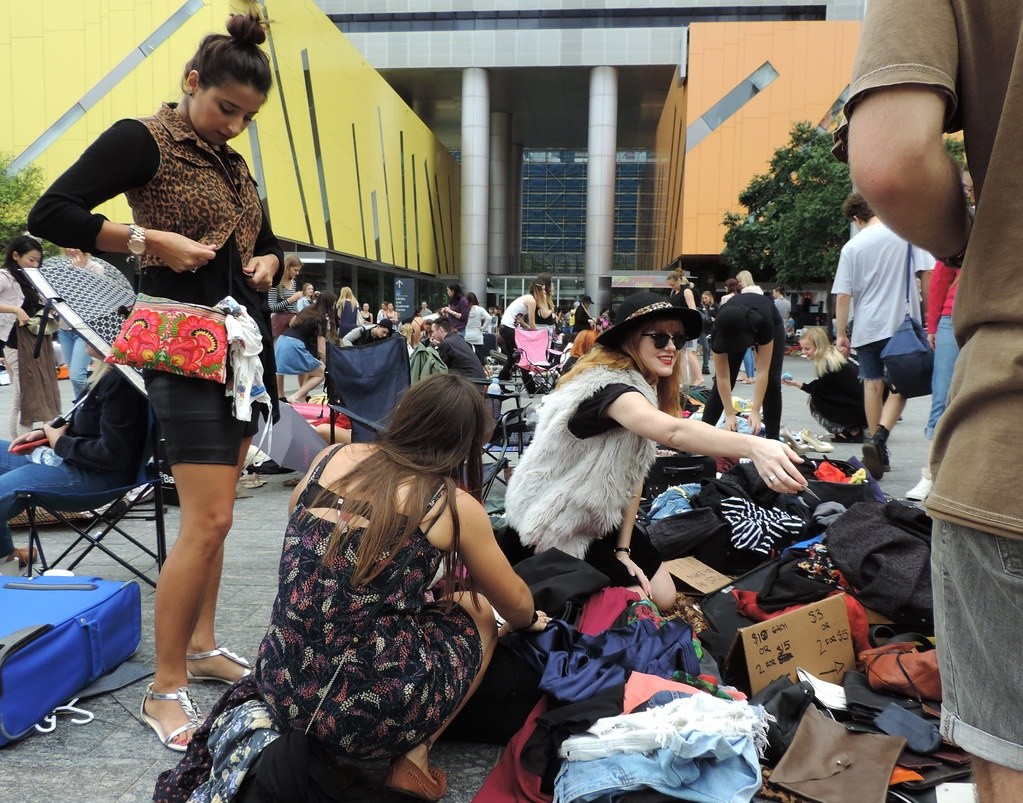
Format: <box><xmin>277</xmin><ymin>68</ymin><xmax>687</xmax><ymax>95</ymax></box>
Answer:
<box><xmin>689</xmin><ymin>384</ymin><xmax>705</xmax><ymax>389</ymax></box>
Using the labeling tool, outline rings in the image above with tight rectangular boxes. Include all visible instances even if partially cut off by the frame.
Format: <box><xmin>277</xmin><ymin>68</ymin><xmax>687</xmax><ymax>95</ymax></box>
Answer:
<box><xmin>769</xmin><ymin>475</ymin><xmax>776</xmax><ymax>483</ymax></box>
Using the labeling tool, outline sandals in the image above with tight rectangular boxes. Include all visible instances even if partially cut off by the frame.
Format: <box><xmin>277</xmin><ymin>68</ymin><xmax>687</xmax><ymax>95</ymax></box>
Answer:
<box><xmin>186</xmin><ymin>647</ymin><xmax>253</xmax><ymax>687</ymax></box>
<box><xmin>140</xmin><ymin>682</ymin><xmax>203</xmax><ymax>751</ymax></box>
<box><xmin>249</xmin><ymin>474</ymin><xmax>266</xmax><ymax>483</ymax></box>
<box><xmin>242</xmin><ymin>476</ymin><xmax>263</xmax><ymax>488</ymax></box>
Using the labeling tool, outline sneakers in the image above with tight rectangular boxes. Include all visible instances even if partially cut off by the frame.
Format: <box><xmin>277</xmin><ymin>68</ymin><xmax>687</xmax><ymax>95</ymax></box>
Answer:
<box><xmin>906</xmin><ymin>477</ymin><xmax>933</xmax><ymax>500</ymax></box>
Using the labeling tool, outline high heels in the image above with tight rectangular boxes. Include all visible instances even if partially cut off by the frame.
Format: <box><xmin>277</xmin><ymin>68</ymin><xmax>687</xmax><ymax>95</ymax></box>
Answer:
<box><xmin>779</xmin><ymin>428</ymin><xmax>864</xmax><ymax>454</ymax></box>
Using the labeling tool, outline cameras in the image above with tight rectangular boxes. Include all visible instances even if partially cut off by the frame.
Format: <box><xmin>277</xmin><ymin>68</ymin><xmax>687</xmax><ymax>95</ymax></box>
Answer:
<box><xmin>49</xmin><ymin>415</ymin><xmax>65</xmax><ymax>428</ymax></box>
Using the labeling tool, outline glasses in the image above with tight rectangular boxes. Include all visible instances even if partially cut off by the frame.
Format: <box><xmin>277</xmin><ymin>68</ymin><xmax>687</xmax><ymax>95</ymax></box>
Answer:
<box><xmin>641</xmin><ymin>332</ymin><xmax>686</xmax><ymax>350</ymax></box>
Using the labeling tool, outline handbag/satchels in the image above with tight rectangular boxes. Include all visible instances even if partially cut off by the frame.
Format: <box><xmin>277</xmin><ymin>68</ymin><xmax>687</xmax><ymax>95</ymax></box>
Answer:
<box><xmin>749</xmin><ymin>674</ymin><xmax>834</xmax><ymax>761</ymax></box>
<box><xmin>859</xmin><ymin>639</ymin><xmax>942</xmax><ymax>700</ymax></box>
<box><xmin>103</xmin><ymin>293</ymin><xmax>228</xmax><ymax>383</ymax></box>
<box><xmin>881</xmin><ymin>314</ymin><xmax>933</xmax><ymax>399</ymax></box>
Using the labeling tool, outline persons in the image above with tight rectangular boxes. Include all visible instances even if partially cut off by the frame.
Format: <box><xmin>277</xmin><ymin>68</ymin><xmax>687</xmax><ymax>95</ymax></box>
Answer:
<box><xmin>0</xmin><ymin>342</ymin><xmax>150</xmax><ymax>577</ymax></box>
<box><xmin>27</xmin><ymin>6</ymin><xmax>285</xmax><ymax>754</ymax></box>
<box><xmin>0</xmin><ymin>236</ymin><xmax>56</xmax><ymax>441</ymax></box>
<box><xmin>843</xmin><ymin>0</ymin><xmax>1023</xmax><ymax>803</ymax></box>
<box><xmin>786</xmin><ymin>311</ymin><xmax>794</xmax><ymax>337</ymax></box>
<box><xmin>701</xmin><ymin>293</ymin><xmax>786</xmax><ymax>441</ymax></box>
<box><xmin>780</xmin><ymin>175</ymin><xmax>985</xmax><ymax>499</ymax></box>
<box><xmin>503</xmin><ymin>291</ymin><xmax>808</xmax><ymax>611</ymax></box>
<box><xmin>267</xmin><ymin>255</ymin><xmax>764</xmax><ymax>404</ymax></box>
<box><xmin>772</xmin><ymin>287</ymin><xmax>791</xmax><ymax>331</ymax></box>
<box><xmin>256</xmin><ymin>372</ymin><xmax>554</xmax><ymax>802</ymax></box>
<box><xmin>58</xmin><ymin>246</ymin><xmax>104</xmax><ymax>400</ymax></box>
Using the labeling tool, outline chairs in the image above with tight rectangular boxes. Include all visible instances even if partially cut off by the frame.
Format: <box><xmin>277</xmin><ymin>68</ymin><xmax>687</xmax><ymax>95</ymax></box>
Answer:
<box><xmin>12</xmin><ymin>401</ymin><xmax>172</xmax><ymax>591</ymax></box>
<box><xmin>322</xmin><ymin>333</ymin><xmax>412</xmax><ymax>448</ymax></box>
<box><xmin>457</xmin><ymin>407</ymin><xmax>528</xmax><ymax>507</ymax></box>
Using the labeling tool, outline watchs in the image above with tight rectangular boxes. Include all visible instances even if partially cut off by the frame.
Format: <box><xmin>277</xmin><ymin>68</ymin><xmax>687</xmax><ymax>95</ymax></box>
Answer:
<box><xmin>528</xmin><ymin>610</ymin><xmax>538</xmax><ymax>626</ymax></box>
<box><xmin>127</xmin><ymin>224</ymin><xmax>146</xmax><ymax>257</ymax></box>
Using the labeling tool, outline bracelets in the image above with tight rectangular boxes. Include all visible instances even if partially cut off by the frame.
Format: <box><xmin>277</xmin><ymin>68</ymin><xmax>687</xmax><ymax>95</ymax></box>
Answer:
<box><xmin>453</xmin><ymin>311</ymin><xmax>456</xmax><ymax>316</ymax></box>
<box><xmin>614</xmin><ymin>547</ymin><xmax>631</xmax><ymax>555</ymax></box>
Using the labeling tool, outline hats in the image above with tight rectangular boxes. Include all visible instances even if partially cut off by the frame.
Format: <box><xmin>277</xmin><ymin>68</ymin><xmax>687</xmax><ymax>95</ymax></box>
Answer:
<box><xmin>596</xmin><ymin>292</ymin><xmax>703</xmax><ymax>351</ymax></box>
<box><xmin>751</xmin><ymin>314</ymin><xmax>774</xmax><ymax>345</ymax></box>
<box><xmin>378</xmin><ymin>319</ymin><xmax>393</xmax><ymax>328</ymax></box>
<box><xmin>579</xmin><ymin>295</ymin><xmax>594</xmax><ymax>304</ymax></box>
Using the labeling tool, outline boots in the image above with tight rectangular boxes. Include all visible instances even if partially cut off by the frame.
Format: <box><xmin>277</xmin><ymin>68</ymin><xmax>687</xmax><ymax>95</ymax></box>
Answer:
<box><xmin>883</xmin><ymin>455</ymin><xmax>891</xmax><ymax>472</ymax></box>
<box><xmin>861</xmin><ymin>424</ymin><xmax>890</xmax><ymax>481</ymax></box>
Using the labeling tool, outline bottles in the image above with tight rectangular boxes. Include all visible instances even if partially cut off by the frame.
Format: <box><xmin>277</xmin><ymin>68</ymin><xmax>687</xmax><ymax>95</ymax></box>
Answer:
<box><xmin>25</xmin><ymin>445</ymin><xmax>63</xmax><ymax>466</ymax></box>
<box><xmin>487</xmin><ymin>378</ymin><xmax>501</xmax><ymax>395</ymax></box>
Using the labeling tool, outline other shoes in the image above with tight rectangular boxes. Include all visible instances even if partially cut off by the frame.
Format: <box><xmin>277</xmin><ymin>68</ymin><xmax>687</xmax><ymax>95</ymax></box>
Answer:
<box><xmin>737</xmin><ymin>372</ymin><xmax>755</xmax><ymax>384</ymax></box>
<box><xmin>529</xmin><ymin>387</ymin><xmax>544</xmax><ymax>394</ymax></box>
<box><xmin>7</xmin><ymin>547</ymin><xmax>37</xmax><ymax>569</ymax></box>
<box><xmin>702</xmin><ymin>369</ymin><xmax>710</xmax><ymax>374</ymax></box>
<box><xmin>501</xmin><ymin>387</ymin><xmax>513</xmax><ymax>394</ymax></box>
<box><xmin>283</xmin><ymin>474</ymin><xmax>303</xmax><ymax>486</ymax></box>
<box><xmin>386</xmin><ymin>756</ymin><xmax>446</xmax><ymax>802</ymax></box>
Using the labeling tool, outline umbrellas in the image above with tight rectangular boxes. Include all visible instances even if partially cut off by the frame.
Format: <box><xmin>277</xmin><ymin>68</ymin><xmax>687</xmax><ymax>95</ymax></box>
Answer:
<box><xmin>16</xmin><ymin>267</ymin><xmax>149</xmax><ymax>401</ymax></box>
